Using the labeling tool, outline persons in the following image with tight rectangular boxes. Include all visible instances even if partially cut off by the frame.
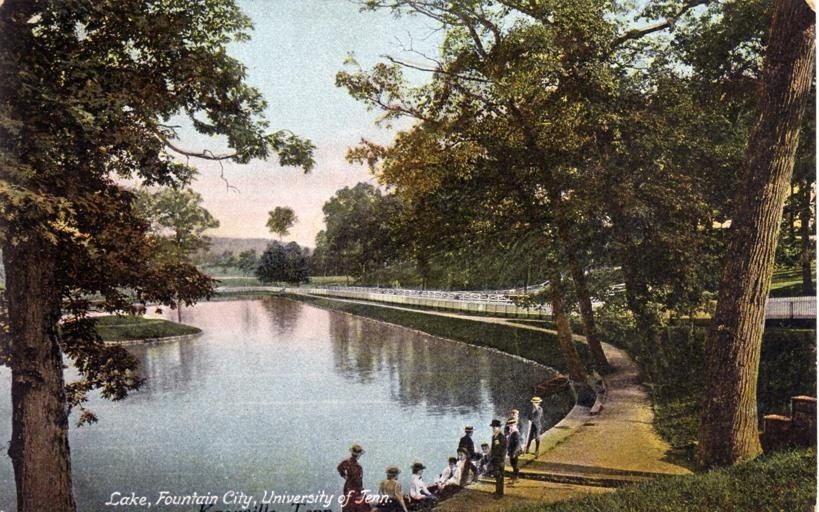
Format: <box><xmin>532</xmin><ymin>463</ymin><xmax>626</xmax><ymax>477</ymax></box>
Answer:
<box><xmin>437</xmin><ymin>456</ymin><xmax>460</xmax><ymax>494</ymax></box>
<box><xmin>506</xmin><ymin>420</ymin><xmax>523</xmax><ymax>487</ymax></box>
<box><xmin>378</xmin><ymin>467</ymin><xmax>408</xmax><ymax>511</ymax></box>
<box><xmin>458</xmin><ymin>426</ymin><xmax>476</xmax><ymax>457</ymax></box>
<box><xmin>488</xmin><ymin>419</ymin><xmax>507</xmax><ymax>500</ymax></box>
<box><xmin>410</xmin><ymin>463</ymin><xmax>438</xmax><ymax>510</ymax></box>
<box><xmin>476</xmin><ymin>442</ymin><xmax>491</xmax><ymax>476</ymax></box>
<box><xmin>525</xmin><ymin>396</ymin><xmax>543</xmax><ymax>459</ymax></box>
<box><xmin>503</xmin><ymin>408</ymin><xmax>521</xmax><ymax>435</ymax></box>
<box><xmin>336</xmin><ymin>444</ymin><xmax>369</xmax><ymax>511</ymax></box>
<box><xmin>457</xmin><ymin>447</ymin><xmax>478</xmax><ymax>488</ymax></box>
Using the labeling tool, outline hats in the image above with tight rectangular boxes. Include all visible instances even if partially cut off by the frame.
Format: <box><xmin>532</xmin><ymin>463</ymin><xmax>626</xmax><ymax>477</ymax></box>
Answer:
<box><xmin>446</xmin><ymin>457</ymin><xmax>458</xmax><ymax>462</ymax></box>
<box><xmin>385</xmin><ymin>466</ymin><xmax>401</xmax><ymax>475</ymax></box>
<box><xmin>463</xmin><ymin>425</ymin><xmax>475</xmax><ymax>431</ymax></box>
<box><xmin>506</xmin><ymin>418</ymin><xmax>517</xmax><ymax>426</ymax></box>
<box><xmin>348</xmin><ymin>444</ymin><xmax>365</xmax><ymax>456</ymax></box>
<box><xmin>457</xmin><ymin>447</ymin><xmax>468</xmax><ymax>454</ymax></box>
<box><xmin>489</xmin><ymin>419</ymin><xmax>502</xmax><ymax>426</ymax></box>
<box><xmin>530</xmin><ymin>397</ymin><xmax>543</xmax><ymax>403</ymax></box>
<box><xmin>411</xmin><ymin>463</ymin><xmax>426</xmax><ymax>469</ymax></box>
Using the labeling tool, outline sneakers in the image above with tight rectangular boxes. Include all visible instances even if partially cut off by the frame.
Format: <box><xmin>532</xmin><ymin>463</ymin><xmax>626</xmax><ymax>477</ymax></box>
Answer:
<box><xmin>526</xmin><ymin>448</ymin><xmax>529</xmax><ymax>453</ymax></box>
<box><xmin>506</xmin><ymin>478</ymin><xmax>521</xmax><ymax>488</ymax></box>
<box><xmin>492</xmin><ymin>491</ymin><xmax>506</xmax><ymax>500</ymax></box>
<box><xmin>533</xmin><ymin>453</ymin><xmax>538</xmax><ymax>458</ymax></box>
